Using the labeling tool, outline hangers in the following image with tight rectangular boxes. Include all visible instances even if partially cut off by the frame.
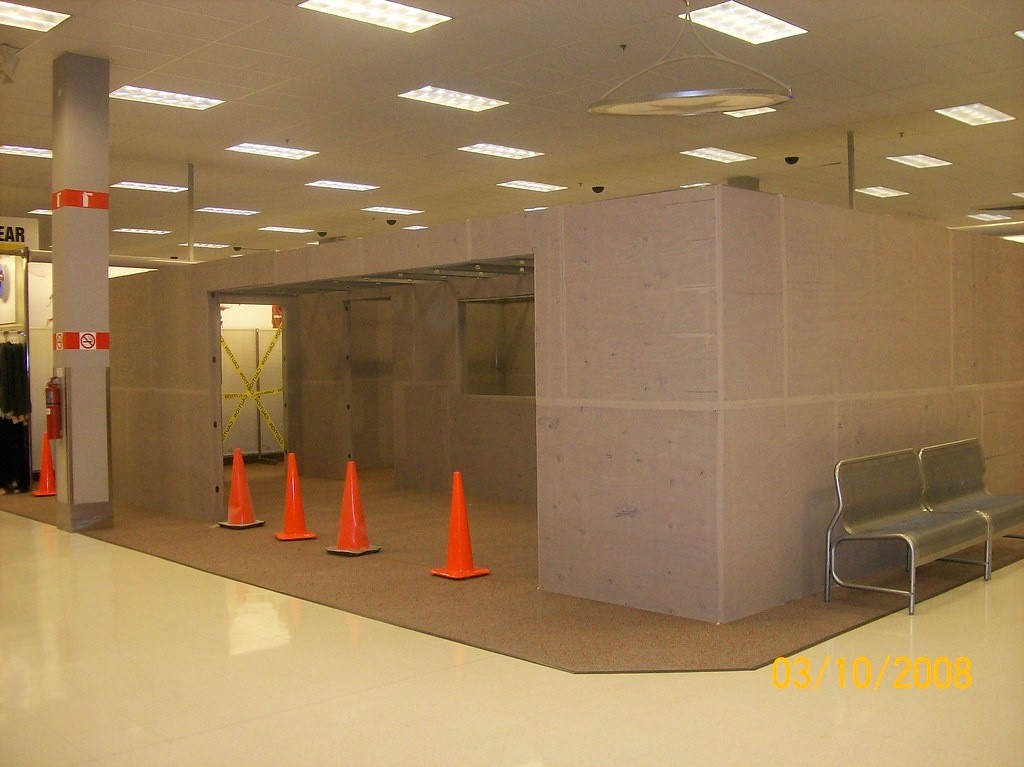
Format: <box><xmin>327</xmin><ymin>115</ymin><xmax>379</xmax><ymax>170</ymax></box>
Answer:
<box><xmin>0</xmin><ymin>327</ymin><xmax>20</xmax><ymax>344</ymax></box>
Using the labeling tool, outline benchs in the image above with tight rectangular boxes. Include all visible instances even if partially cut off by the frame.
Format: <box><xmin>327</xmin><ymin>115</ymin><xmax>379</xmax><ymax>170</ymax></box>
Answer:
<box><xmin>824</xmin><ymin>436</ymin><xmax>990</xmax><ymax>611</ymax></box>
<box><xmin>918</xmin><ymin>437</ymin><xmax>1024</xmax><ymax>580</ymax></box>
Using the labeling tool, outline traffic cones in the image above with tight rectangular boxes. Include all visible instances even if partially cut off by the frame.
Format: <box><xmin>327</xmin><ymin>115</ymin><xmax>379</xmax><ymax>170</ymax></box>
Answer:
<box><xmin>29</xmin><ymin>431</ymin><xmax>57</xmax><ymax>497</ymax></box>
<box><xmin>324</xmin><ymin>461</ymin><xmax>382</xmax><ymax>558</ymax></box>
<box><xmin>427</xmin><ymin>472</ymin><xmax>492</xmax><ymax>580</ymax></box>
<box><xmin>272</xmin><ymin>451</ymin><xmax>319</xmax><ymax>542</ymax></box>
<box><xmin>215</xmin><ymin>448</ymin><xmax>267</xmax><ymax>530</ymax></box>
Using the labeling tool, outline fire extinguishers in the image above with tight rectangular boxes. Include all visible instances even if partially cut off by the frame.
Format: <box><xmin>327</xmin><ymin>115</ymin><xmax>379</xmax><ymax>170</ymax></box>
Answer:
<box><xmin>43</xmin><ymin>375</ymin><xmax>62</xmax><ymax>440</ymax></box>
